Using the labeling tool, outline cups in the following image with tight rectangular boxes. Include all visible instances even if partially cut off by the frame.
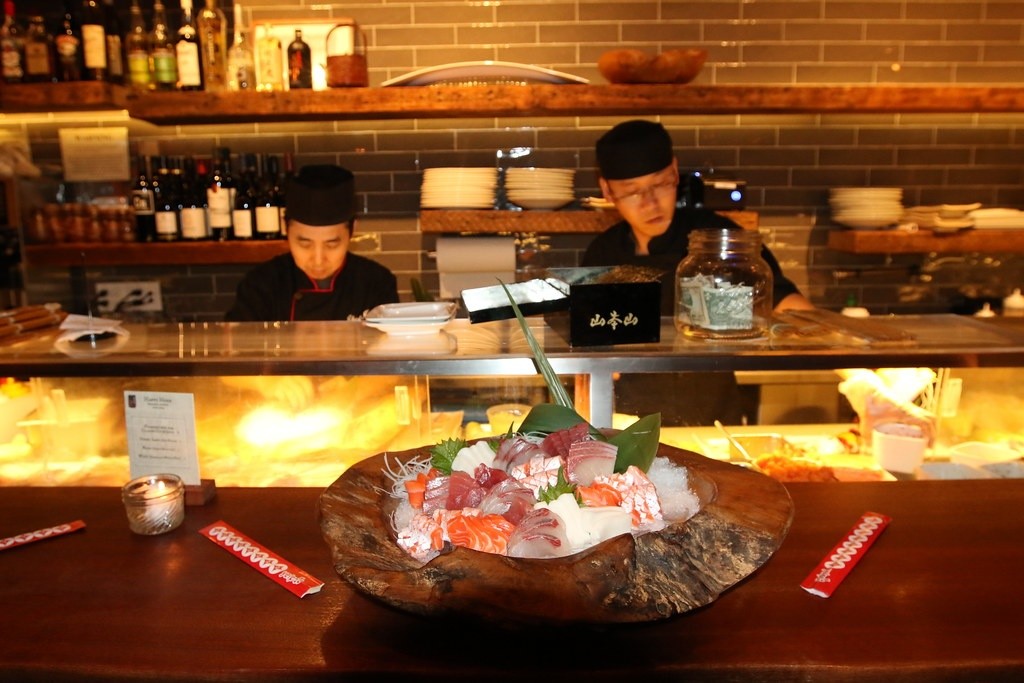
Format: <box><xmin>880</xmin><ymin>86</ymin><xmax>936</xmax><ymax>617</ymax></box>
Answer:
<box><xmin>121</xmin><ymin>474</ymin><xmax>186</xmax><ymax>535</ymax></box>
<box><xmin>871</xmin><ymin>426</ymin><xmax>928</xmax><ymax>474</ymax></box>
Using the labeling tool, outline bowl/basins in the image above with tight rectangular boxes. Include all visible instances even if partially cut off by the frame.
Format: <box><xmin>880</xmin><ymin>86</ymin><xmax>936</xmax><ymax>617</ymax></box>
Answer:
<box><xmin>598</xmin><ymin>47</ymin><xmax>707</xmax><ymax>84</ymax></box>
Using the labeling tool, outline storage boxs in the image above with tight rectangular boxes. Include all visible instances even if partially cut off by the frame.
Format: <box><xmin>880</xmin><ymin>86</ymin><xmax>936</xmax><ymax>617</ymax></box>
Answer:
<box><xmin>542</xmin><ymin>265</ymin><xmax>661</xmax><ymax>350</ymax></box>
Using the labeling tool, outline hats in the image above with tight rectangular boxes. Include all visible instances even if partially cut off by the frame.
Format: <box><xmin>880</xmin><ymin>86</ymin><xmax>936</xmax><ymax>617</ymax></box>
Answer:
<box><xmin>287</xmin><ymin>166</ymin><xmax>355</xmax><ymax>227</ymax></box>
<box><xmin>596</xmin><ymin>119</ymin><xmax>673</xmax><ymax>180</ymax></box>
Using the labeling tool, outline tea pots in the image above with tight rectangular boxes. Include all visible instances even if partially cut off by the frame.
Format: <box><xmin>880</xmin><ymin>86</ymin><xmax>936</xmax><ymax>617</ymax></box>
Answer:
<box><xmin>320</xmin><ymin>23</ymin><xmax>369</xmax><ymax>88</ymax></box>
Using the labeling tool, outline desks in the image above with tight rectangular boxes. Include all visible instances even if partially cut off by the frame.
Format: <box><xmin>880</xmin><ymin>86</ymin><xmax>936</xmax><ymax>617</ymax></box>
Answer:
<box><xmin>0</xmin><ymin>481</ymin><xmax>1024</xmax><ymax>683</ymax></box>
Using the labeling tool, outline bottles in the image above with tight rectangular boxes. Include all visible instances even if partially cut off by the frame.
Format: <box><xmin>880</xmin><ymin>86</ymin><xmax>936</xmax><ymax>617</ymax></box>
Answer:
<box><xmin>674</xmin><ymin>228</ymin><xmax>774</xmax><ymax>340</ymax></box>
<box><xmin>1</xmin><ymin>0</ymin><xmax>312</xmax><ymax>91</ymax></box>
<box><xmin>27</xmin><ymin>146</ymin><xmax>297</xmax><ymax>243</ymax></box>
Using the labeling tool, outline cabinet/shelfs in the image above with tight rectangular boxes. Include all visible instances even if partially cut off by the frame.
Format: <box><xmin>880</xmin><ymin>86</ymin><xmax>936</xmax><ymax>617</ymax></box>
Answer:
<box><xmin>0</xmin><ymin>80</ymin><xmax>1024</xmax><ymax>268</ymax></box>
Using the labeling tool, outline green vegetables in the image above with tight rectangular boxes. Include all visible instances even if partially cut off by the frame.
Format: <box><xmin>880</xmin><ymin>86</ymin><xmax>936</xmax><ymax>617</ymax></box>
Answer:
<box><xmin>538</xmin><ymin>465</ymin><xmax>586</xmax><ymax>508</ymax></box>
<box><xmin>429</xmin><ymin>437</ymin><xmax>467</xmax><ymax>475</ymax></box>
<box><xmin>488</xmin><ymin>421</ymin><xmax>514</xmax><ymax>452</ymax></box>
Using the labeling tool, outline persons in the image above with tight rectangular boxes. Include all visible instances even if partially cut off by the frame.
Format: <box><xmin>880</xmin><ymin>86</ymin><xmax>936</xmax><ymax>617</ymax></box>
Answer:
<box><xmin>576</xmin><ymin>120</ymin><xmax>815</xmax><ymax>312</ymax></box>
<box><xmin>223</xmin><ymin>162</ymin><xmax>398</xmax><ymax>323</ymax></box>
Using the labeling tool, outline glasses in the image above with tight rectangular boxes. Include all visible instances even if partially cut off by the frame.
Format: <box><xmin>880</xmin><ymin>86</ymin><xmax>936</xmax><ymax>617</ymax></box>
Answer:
<box><xmin>609</xmin><ymin>170</ymin><xmax>679</xmax><ymax>204</ymax></box>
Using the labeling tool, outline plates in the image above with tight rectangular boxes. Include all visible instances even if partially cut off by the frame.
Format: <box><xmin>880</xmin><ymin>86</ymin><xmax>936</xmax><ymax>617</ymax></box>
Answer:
<box><xmin>365</xmin><ymin>302</ymin><xmax>460</xmax><ymax>335</ymax></box>
<box><xmin>419</xmin><ymin>167</ymin><xmax>576</xmax><ymax>212</ymax></box>
<box><xmin>828</xmin><ymin>188</ymin><xmax>1024</xmax><ymax>232</ymax></box>
